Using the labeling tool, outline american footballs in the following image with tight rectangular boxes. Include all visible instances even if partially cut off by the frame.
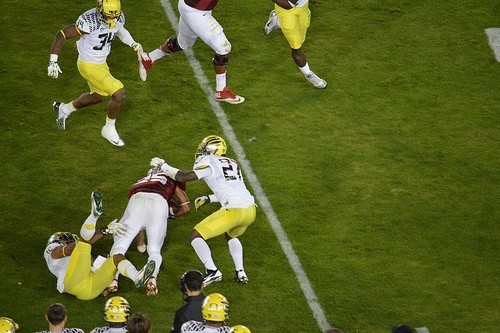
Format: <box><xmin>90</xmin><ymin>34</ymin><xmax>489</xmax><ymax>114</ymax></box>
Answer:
<box><xmin>169</xmin><ymin>199</ymin><xmax>179</xmax><ymax>215</ymax></box>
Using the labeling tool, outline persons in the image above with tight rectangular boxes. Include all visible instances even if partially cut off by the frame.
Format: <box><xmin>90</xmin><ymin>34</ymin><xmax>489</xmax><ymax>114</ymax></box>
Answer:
<box><xmin>265</xmin><ymin>0</ymin><xmax>328</xmax><ymax>89</ymax></box>
<box><xmin>48</xmin><ymin>0</ymin><xmax>144</xmax><ymax>146</ymax></box>
<box><xmin>0</xmin><ymin>270</ymin><xmax>415</xmax><ymax>333</ymax></box>
<box><xmin>44</xmin><ymin>189</ymin><xmax>155</xmax><ymax>300</ymax></box>
<box><xmin>101</xmin><ymin>167</ymin><xmax>192</xmax><ymax>297</ymax></box>
<box><xmin>149</xmin><ymin>135</ymin><xmax>257</xmax><ymax>288</ymax></box>
<box><xmin>138</xmin><ymin>0</ymin><xmax>245</xmax><ymax>104</ymax></box>
